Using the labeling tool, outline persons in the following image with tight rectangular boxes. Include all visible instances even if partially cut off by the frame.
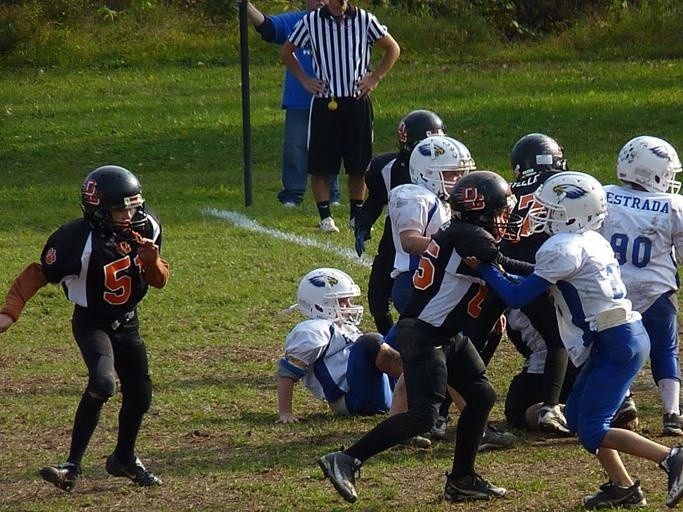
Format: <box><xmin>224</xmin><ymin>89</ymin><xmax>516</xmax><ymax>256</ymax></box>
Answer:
<box><xmin>271</xmin><ymin>110</ymin><xmax>683</xmax><ymax>449</ymax></box>
<box><xmin>1</xmin><ymin>165</ymin><xmax>171</xmax><ymax>493</ymax></box>
<box><xmin>317</xmin><ymin>172</ymin><xmax>536</xmax><ymax>501</ymax></box>
<box><xmin>465</xmin><ymin>172</ymin><xmax>682</xmax><ymax>511</ymax></box>
<box><xmin>249</xmin><ymin>1</ymin><xmax>345</xmax><ymax>211</ymax></box>
<box><xmin>279</xmin><ymin>4</ymin><xmax>401</xmax><ymax>234</ymax></box>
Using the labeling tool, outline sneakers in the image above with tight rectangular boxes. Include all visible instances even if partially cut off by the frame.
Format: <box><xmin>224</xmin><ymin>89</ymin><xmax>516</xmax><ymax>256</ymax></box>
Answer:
<box><xmin>40</xmin><ymin>462</ymin><xmax>82</xmax><ymax>491</ymax></box>
<box><xmin>401</xmin><ymin>415</ymin><xmax>447</xmax><ymax>448</ymax></box>
<box><xmin>477</xmin><ymin>424</ymin><xmax>515</xmax><ymax>450</ymax></box>
<box><xmin>445</xmin><ymin>470</ymin><xmax>506</xmax><ymax>500</ymax></box>
<box><xmin>583</xmin><ymin>397</ymin><xmax>683</xmax><ymax>508</ymax></box>
<box><xmin>537</xmin><ymin>405</ymin><xmax>574</xmax><ymax>437</ymax></box>
<box><xmin>319</xmin><ymin>217</ymin><xmax>339</xmax><ymax>233</ymax></box>
<box><xmin>106</xmin><ymin>454</ymin><xmax>162</xmax><ymax>486</ymax></box>
<box><xmin>317</xmin><ymin>446</ymin><xmax>362</xmax><ymax>503</ymax></box>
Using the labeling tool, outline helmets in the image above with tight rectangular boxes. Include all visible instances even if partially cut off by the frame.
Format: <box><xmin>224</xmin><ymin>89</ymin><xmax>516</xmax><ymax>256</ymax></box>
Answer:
<box><xmin>81</xmin><ymin>165</ymin><xmax>148</xmax><ymax>232</ymax></box>
<box><xmin>399</xmin><ymin>110</ymin><xmax>683</xmax><ymax>243</ymax></box>
<box><xmin>297</xmin><ymin>268</ymin><xmax>363</xmax><ymax>326</ymax></box>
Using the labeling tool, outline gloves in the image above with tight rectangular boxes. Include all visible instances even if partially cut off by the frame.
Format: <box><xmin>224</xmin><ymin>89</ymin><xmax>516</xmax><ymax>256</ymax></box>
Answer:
<box><xmin>355</xmin><ymin>229</ymin><xmax>371</xmax><ymax>257</ymax></box>
